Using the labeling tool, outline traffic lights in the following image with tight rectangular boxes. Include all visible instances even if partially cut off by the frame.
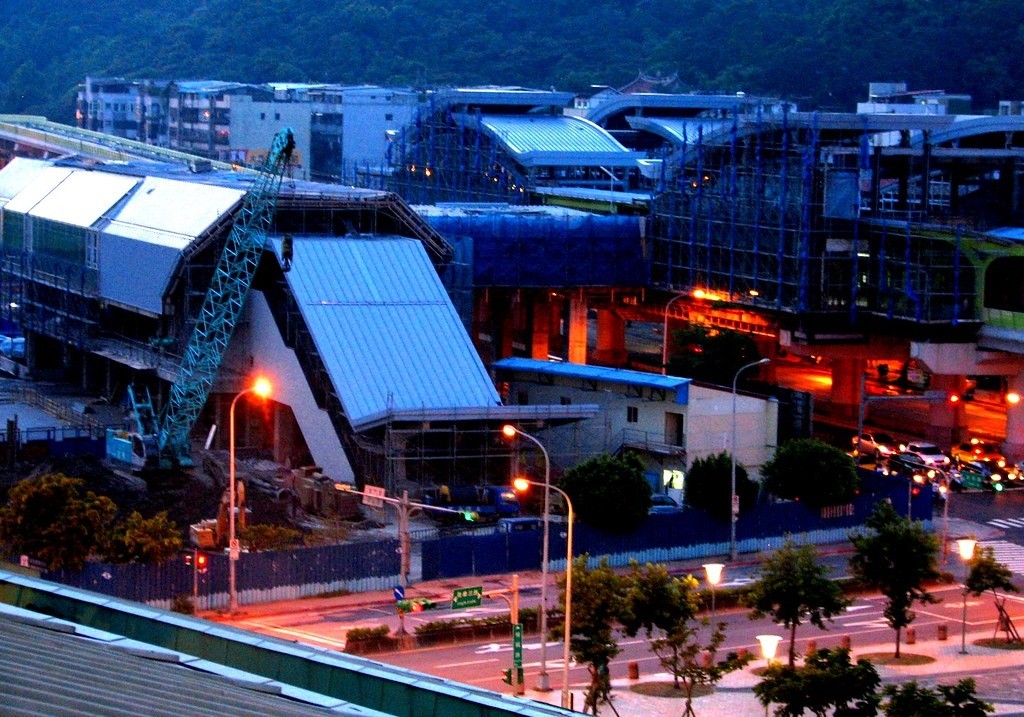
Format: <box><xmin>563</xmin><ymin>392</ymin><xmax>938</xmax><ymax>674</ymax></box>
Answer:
<box><xmin>197</xmin><ymin>556</ymin><xmax>205</xmax><ymax>569</ymax></box>
<box><xmin>950</xmin><ymin>394</ymin><xmax>958</xmax><ymax>403</ymax></box>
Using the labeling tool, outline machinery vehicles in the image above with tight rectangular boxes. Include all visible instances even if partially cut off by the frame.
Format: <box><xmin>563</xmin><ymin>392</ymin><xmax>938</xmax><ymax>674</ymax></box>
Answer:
<box><xmin>190</xmin><ymin>482</ymin><xmax>249</xmax><ymax>549</ymax></box>
<box><xmin>105</xmin><ymin>127</ymin><xmax>299</xmax><ymax>490</ymax></box>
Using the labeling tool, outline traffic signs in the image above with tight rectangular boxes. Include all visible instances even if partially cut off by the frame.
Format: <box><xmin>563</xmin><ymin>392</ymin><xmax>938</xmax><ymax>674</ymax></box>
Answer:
<box><xmin>450</xmin><ymin>585</ymin><xmax>483</xmax><ymax>609</ymax></box>
<box><xmin>962</xmin><ymin>471</ymin><xmax>989</xmax><ymax>491</ymax></box>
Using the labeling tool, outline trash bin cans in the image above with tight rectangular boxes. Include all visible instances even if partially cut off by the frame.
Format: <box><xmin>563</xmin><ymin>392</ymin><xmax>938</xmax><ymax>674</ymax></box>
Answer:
<box><xmin>629</xmin><ymin>662</ymin><xmax>639</xmax><ymax>680</ymax></box>
<box><xmin>806</xmin><ymin>640</ymin><xmax>816</xmax><ymax>656</ymax></box>
<box><xmin>938</xmin><ymin>625</ymin><xmax>947</xmax><ymax>640</ymax></box>
<box><xmin>737</xmin><ymin>648</ymin><xmax>748</xmax><ymax>660</ymax></box>
<box><xmin>906</xmin><ymin>627</ymin><xmax>915</xmax><ymax>644</ymax></box>
<box><xmin>840</xmin><ymin>636</ymin><xmax>851</xmax><ymax>649</ymax></box>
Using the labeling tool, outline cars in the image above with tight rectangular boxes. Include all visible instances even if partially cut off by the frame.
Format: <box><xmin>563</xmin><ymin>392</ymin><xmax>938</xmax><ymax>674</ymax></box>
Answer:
<box><xmin>886</xmin><ymin>451</ymin><xmax>925</xmax><ymax>475</ymax></box>
<box><xmin>930</xmin><ymin>460</ymin><xmax>1024</xmax><ymax>494</ymax></box>
<box><xmin>851</xmin><ymin>430</ymin><xmax>901</xmax><ymax>460</ymax></box>
<box><xmin>949</xmin><ymin>441</ymin><xmax>1006</xmax><ymax>469</ymax></box>
<box><xmin>906</xmin><ymin>440</ymin><xmax>951</xmax><ymax>469</ymax></box>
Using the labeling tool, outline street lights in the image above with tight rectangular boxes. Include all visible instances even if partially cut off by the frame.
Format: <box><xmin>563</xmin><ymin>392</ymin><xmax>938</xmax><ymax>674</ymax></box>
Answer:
<box><xmin>660</xmin><ymin>289</ymin><xmax>706</xmax><ymax>376</ymax></box>
<box><xmin>730</xmin><ymin>358</ymin><xmax>772</xmax><ymax>561</ymax></box>
<box><xmin>701</xmin><ymin>563</ymin><xmax>725</xmax><ymax>642</ymax></box>
<box><xmin>229</xmin><ymin>379</ymin><xmax>273</xmax><ymax>614</ymax></box>
<box><xmin>514</xmin><ymin>477</ymin><xmax>574</xmax><ymax>712</ymax></box>
<box><xmin>755</xmin><ymin>633</ymin><xmax>783</xmax><ymax>717</ymax></box>
<box><xmin>955</xmin><ymin>538</ymin><xmax>977</xmax><ymax>653</ymax></box>
<box><xmin>503</xmin><ymin>424</ymin><xmax>550</xmax><ymax>691</ymax></box>
